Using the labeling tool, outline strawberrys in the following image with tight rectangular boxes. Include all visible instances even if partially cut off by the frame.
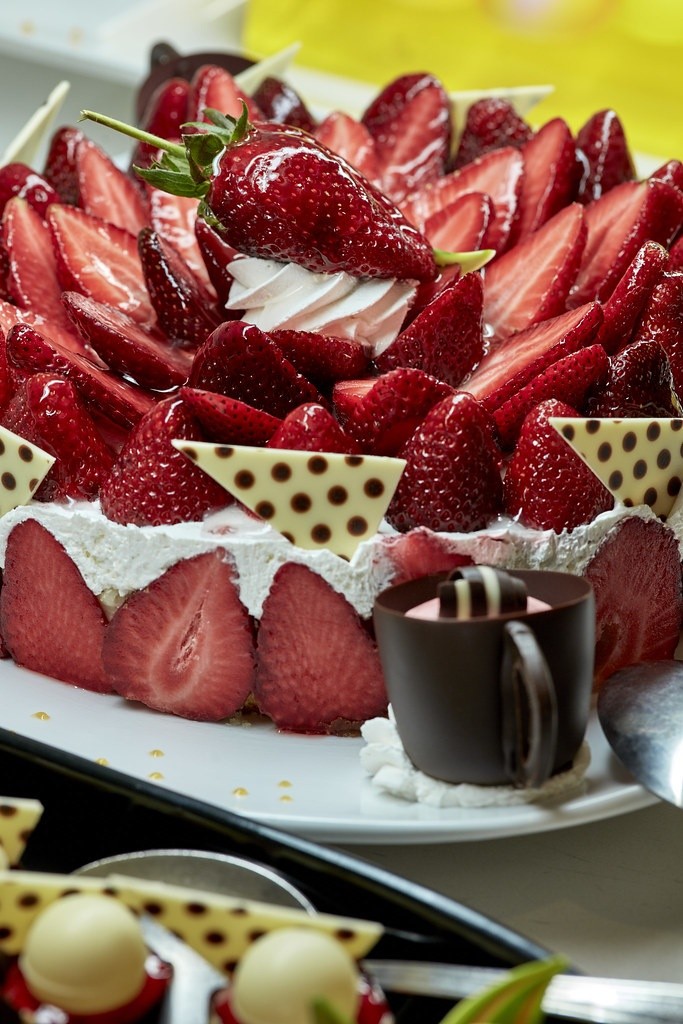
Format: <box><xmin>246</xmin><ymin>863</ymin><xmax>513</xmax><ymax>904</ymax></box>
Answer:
<box><xmin>0</xmin><ymin>64</ymin><xmax>683</xmax><ymax>736</ymax></box>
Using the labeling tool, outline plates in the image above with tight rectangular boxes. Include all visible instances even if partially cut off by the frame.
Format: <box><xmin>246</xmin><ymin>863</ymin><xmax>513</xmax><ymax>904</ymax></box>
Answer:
<box><xmin>0</xmin><ymin>722</ymin><xmax>590</xmax><ymax>1024</ymax></box>
<box><xmin>0</xmin><ymin>642</ymin><xmax>683</xmax><ymax>859</ymax></box>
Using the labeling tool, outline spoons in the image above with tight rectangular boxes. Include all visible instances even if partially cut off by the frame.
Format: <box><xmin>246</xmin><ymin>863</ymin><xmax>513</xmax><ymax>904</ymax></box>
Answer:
<box><xmin>61</xmin><ymin>849</ymin><xmax>683</xmax><ymax>1023</ymax></box>
<box><xmin>597</xmin><ymin>655</ymin><xmax>683</xmax><ymax>812</ymax></box>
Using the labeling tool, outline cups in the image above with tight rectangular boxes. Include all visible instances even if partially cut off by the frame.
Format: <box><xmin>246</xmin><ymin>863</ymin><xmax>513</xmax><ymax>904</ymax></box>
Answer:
<box><xmin>365</xmin><ymin>566</ymin><xmax>603</xmax><ymax>791</ymax></box>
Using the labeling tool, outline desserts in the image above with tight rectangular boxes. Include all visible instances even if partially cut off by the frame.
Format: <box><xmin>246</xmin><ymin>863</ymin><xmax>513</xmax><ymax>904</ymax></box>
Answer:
<box><xmin>17</xmin><ymin>888</ymin><xmax>361</xmax><ymax>1024</ymax></box>
